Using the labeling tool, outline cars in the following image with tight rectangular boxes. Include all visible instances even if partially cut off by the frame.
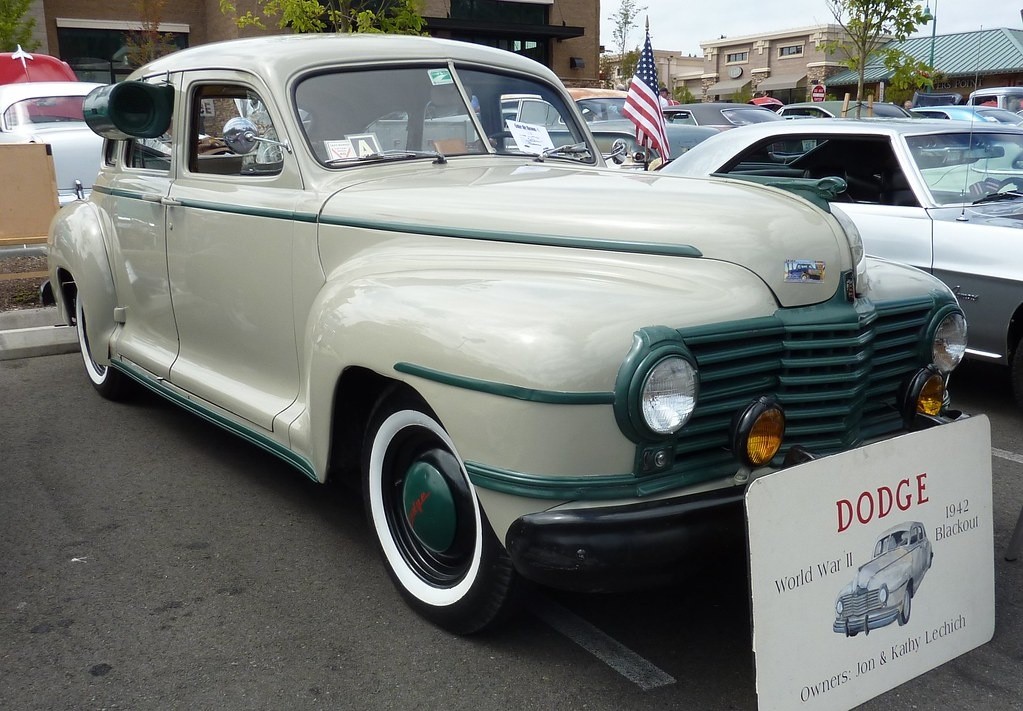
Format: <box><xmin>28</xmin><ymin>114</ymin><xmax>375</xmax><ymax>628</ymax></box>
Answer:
<box><xmin>909</xmin><ymin>105</ymin><xmax>1023</xmax><ymax>194</ymax></box>
<box><xmin>661</xmin><ymin>102</ymin><xmax>826</xmax><ymax>159</ymax></box>
<box><xmin>776</xmin><ymin>101</ymin><xmax>912</xmax><ymax>119</ymax></box>
<box><xmin>658</xmin><ymin>116</ymin><xmax>1023</xmax><ymax>408</ymax></box>
<box><xmin>500</xmin><ymin>87</ymin><xmax>721</xmax><ymax>170</ymax></box>
<box><xmin>1</xmin><ymin>44</ymin><xmax>173</xmax><ymax>208</ymax></box>
<box><xmin>745</xmin><ymin>97</ymin><xmax>784</xmax><ymax>112</ymax></box>
<box><xmin>832</xmin><ymin>521</ymin><xmax>933</xmax><ymax>638</ymax></box>
<box><xmin>967</xmin><ymin>87</ymin><xmax>1023</xmax><ymax>116</ymax></box>
<box><xmin>47</xmin><ymin>32</ymin><xmax>974</xmax><ymax>641</ymax></box>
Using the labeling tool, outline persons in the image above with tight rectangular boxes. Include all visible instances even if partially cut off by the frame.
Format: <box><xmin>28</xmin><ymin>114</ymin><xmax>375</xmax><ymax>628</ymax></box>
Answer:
<box><xmin>659</xmin><ymin>87</ymin><xmax>669</xmax><ymax>99</ymax></box>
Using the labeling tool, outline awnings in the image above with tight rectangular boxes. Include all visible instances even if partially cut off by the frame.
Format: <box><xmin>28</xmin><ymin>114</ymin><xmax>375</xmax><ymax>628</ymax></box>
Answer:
<box><xmin>707</xmin><ymin>80</ymin><xmax>752</xmax><ymax>95</ymax></box>
<box><xmin>757</xmin><ymin>73</ymin><xmax>807</xmax><ymax>91</ymax></box>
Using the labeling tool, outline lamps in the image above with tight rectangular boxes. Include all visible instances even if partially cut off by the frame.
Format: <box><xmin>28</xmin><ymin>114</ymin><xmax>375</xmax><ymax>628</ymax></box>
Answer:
<box><xmin>570</xmin><ymin>56</ymin><xmax>585</xmax><ymax>71</ymax></box>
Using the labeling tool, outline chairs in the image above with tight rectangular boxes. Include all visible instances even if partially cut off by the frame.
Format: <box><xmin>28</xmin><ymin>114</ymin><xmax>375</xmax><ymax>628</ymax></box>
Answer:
<box><xmin>432</xmin><ymin>137</ymin><xmax>467</xmax><ymax>155</ymax></box>
<box><xmin>844</xmin><ymin>148</ymin><xmax>918</xmax><ymax>207</ymax></box>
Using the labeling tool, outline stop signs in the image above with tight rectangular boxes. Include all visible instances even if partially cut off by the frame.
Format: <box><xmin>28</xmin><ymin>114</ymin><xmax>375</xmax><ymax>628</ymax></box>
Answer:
<box><xmin>812</xmin><ymin>86</ymin><xmax>826</xmax><ymax>102</ymax></box>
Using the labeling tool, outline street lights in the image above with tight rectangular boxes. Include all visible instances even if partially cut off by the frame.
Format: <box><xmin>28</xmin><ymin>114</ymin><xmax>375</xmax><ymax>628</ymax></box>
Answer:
<box><xmin>921</xmin><ymin>0</ymin><xmax>937</xmax><ymax>94</ymax></box>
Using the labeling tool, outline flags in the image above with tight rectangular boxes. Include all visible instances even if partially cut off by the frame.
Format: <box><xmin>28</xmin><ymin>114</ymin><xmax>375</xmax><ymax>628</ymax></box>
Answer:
<box><xmin>621</xmin><ymin>32</ymin><xmax>670</xmax><ymax>165</ymax></box>
